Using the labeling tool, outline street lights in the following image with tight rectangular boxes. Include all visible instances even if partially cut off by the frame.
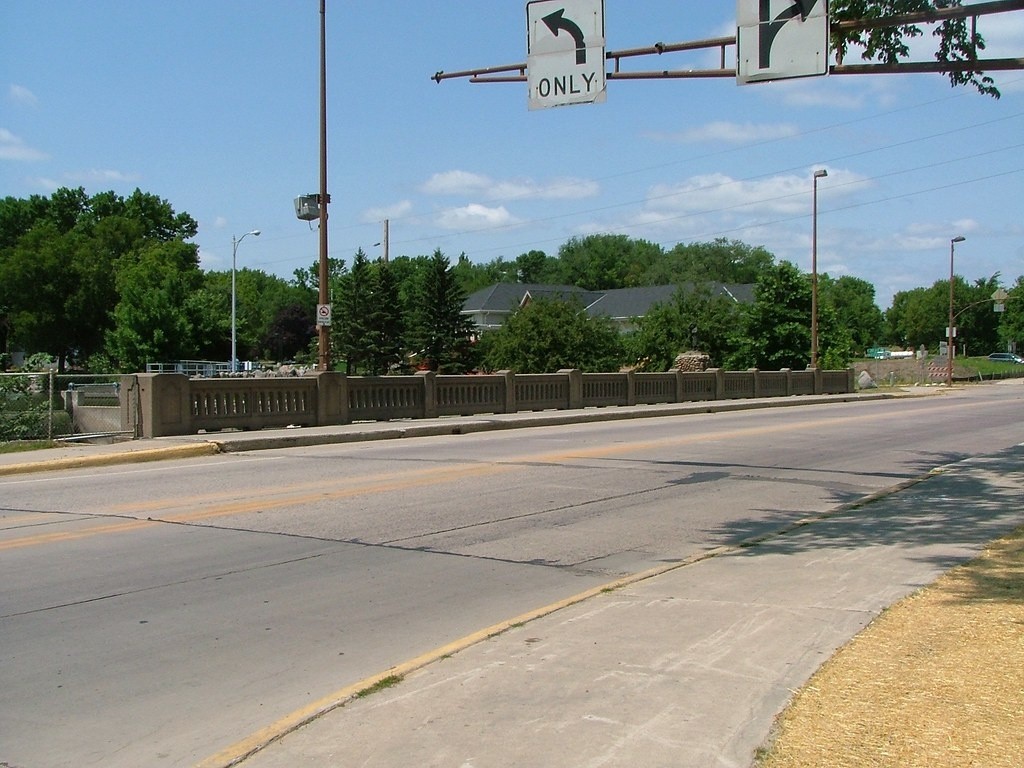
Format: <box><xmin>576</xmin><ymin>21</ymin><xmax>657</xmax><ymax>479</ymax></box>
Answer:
<box><xmin>230</xmin><ymin>229</ymin><xmax>262</xmax><ymax>374</ymax></box>
<box><xmin>372</xmin><ymin>241</ymin><xmax>390</xmax><ymax>264</ymax></box>
<box><xmin>810</xmin><ymin>169</ymin><xmax>828</xmax><ymax>369</ymax></box>
<box><xmin>947</xmin><ymin>236</ymin><xmax>966</xmax><ymax>387</ymax></box>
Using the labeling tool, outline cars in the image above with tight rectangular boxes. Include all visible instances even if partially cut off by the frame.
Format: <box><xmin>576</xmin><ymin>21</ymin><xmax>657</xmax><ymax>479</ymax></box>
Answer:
<box><xmin>987</xmin><ymin>353</ymin><xmax>1024</xmax><ymax>365</ymax></box>
